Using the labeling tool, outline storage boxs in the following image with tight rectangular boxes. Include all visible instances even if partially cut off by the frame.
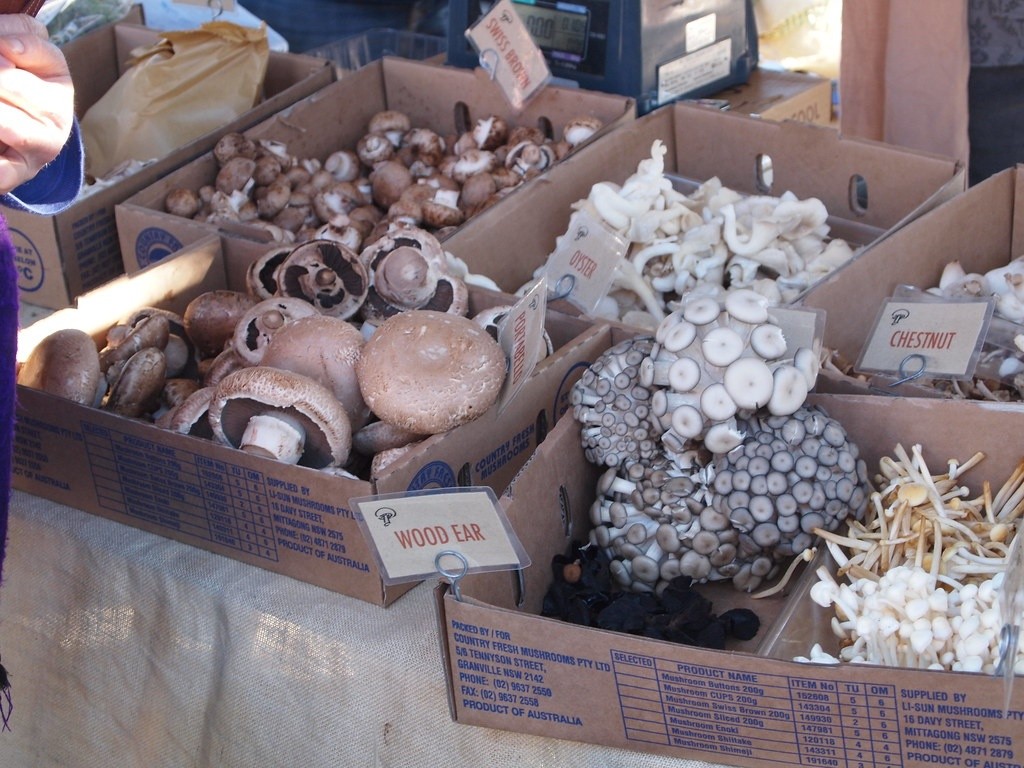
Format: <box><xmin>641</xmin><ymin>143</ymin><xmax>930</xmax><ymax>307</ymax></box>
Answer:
<box><xmin>438</xmin><ymin>102</ymin><xmax>968</xmax><ymax>348</ymax></box>
<box><xmin>0</xmin><ymin>51</ymin><xmax>338</xmax><ymax>308</ymax></box>
<box><xmin>115</xmin><ymin>55</ymin><xmax>634</xmax><ymax>277</ymax></box>
<box><xmin>797</xmin><ymin>161</ymin><xmax>1024</xmax><ymax>403</ymax></box>
<box><xmin>5</xmin><ymin>232</ymin><xmax>610</xmax><ymax>609</ymax></box>
<box><xmin>432</xmin><ymin>394</ymin><xmax>1024</xmax><ymax>768</ymax></box>
<box><xmin>710</xmin><ymin>66</ymin><xmax>833</xmax><ymax>128</ymax></box>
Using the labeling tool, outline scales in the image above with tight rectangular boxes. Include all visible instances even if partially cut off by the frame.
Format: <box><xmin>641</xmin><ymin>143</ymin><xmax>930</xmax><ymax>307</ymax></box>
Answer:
<box><xmin>443</xmin><ymin>0</ymin><xmax>759</xmax><ymax>117</ymax></box>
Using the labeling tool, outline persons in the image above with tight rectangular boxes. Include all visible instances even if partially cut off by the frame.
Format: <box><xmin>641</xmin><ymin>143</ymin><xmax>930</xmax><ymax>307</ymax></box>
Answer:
<box><xmin>0</xmin><ymin>13</ymin><xmax>85</xmax><ymax>590</ymax></box>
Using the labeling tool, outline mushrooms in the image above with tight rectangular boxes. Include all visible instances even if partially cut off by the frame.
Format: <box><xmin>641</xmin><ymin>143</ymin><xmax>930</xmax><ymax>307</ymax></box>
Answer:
<box><xmin>10</xmin><ymin>226</ymin><xmax>549</xmax><ymax>480</ymax></box>
<box><xmin>167</xmin><ymin>108</ymin><xmax>606</xmax><ymax>241</ymax></box>
<box><xmin>553</xmin><ymin>138</ymin><xmax>1024</xmax><ymax>685</ymax></box>
